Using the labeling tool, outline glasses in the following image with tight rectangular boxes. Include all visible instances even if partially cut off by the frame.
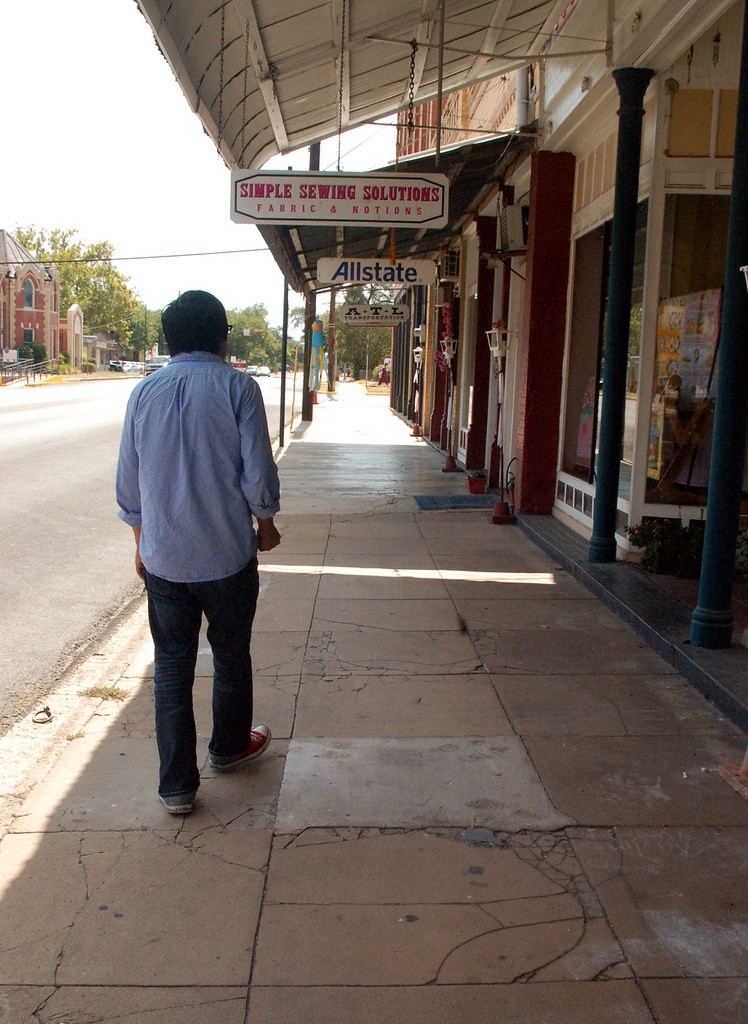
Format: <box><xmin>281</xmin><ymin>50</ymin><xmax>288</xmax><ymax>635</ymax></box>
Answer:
<box><xmin>226</xmin><ymin>324</ymin><xmax>233</xmax><ymax>334</ymax></box>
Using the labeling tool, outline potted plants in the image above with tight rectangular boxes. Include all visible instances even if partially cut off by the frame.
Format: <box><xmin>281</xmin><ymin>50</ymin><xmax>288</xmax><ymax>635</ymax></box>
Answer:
<box><xmin>466</xmin><ymin>469</ymin><xmax>488</xmax><ymax>493</ymax></box>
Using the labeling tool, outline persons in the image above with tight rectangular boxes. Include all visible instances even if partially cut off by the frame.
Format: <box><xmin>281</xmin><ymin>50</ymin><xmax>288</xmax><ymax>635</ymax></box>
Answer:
<box><xmin>378</xmin><ymin>363</ymin><xmax>388</xmax><ymax>386</ymax></box>
<box><xmin>115</xmin><ymin>290</ymin><xmax>282</xmax><ymax>812</ymax></box>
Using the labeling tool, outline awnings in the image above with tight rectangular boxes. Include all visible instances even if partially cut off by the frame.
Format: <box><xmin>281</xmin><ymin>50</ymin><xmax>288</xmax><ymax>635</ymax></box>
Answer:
<box><xmin>134</xmin><ymin>0</ymin><xmax>578</xmax><ymax>171</ymax></box>
<box><xmin>254</xmin><ymin>120</ymin><xmax>538</xmax><ymax>293</ymax></box>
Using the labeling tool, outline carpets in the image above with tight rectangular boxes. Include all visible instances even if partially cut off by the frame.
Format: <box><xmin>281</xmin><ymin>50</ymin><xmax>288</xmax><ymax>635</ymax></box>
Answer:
<box><xmin>413</xmin><ymin>494</ymin><xmax>496</xmax><ymax>510</ymax></box>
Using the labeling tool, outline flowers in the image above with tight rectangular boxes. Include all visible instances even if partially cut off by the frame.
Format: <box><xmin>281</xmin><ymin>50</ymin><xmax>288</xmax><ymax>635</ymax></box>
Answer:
<box><xmin>623</xmin><ymin>518</ymin><xmax>699</xmax><ymax>574</ymax></box>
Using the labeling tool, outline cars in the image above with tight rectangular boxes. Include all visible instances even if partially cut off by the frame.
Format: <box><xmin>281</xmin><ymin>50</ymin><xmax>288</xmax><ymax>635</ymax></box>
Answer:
<box><xmin>246</xmin><ymin>366</ymin><xmax>257</xmax><ymax>376</ymax></box>
<box><xmin>257</xmin><ymin>367</ymin><xmax>271</xmax><ymax>377</ymax></box>
<box><xmin>124</xmin><ymin>355</ymin><xmax>172</xmax><ymax>377</ymax></box>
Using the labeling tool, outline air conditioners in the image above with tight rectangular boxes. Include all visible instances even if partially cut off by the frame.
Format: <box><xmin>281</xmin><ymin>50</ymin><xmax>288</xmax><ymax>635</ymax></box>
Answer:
<box><xmin>499</xmin><ymin>205</ymin><xmax>524</xmax><ymax>251</ymax></box>
<box><xmin>440</xmin><ymin>256</ymin><xmax>459</xmax><ymax>283</ymax></box>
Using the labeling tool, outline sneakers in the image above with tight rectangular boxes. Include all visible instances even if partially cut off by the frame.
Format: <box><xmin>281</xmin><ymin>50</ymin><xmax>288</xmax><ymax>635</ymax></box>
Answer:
<box><xmin>158</xmin><ymin>789</ymin><xmax>195</xmax><ymax>814</ymax></box>
<box><xmin>209</xmin><ymin>725</ymin><xmax>271</xmax><ymax>769</ymax></box>
